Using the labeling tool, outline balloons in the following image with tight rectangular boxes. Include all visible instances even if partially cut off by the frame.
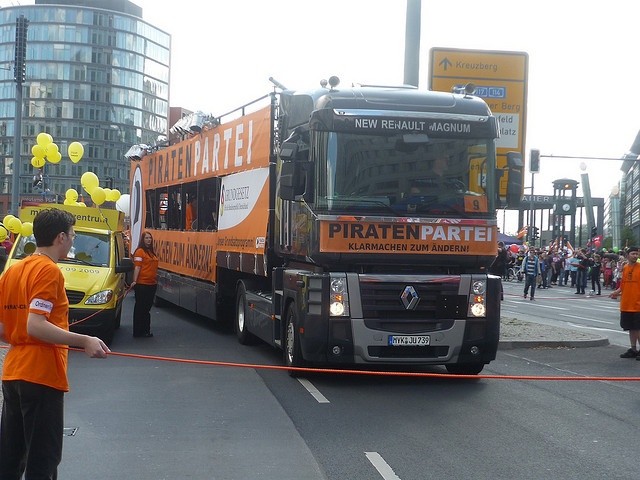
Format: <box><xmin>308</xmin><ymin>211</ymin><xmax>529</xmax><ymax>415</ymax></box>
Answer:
<box><xmin>30</xmin><ymin>129</ymin><xmax>63</xmax><ymax>168</ymax></box>
<box><xmin>63</xmin><ymin>187</ymin><xmax>79</xmax><ymax>202</ymax></box>
<box><xmin>104</xmin><ymin>188</ymin><xmax>111</xmax><ymax>202</ymax></box>
<box><xmin>80</xmin><ymin>172</ymin><xmax>100</xmax><ymax>194</ymax></box>
<box><xmin>109</xmin><ymin>189</ymin><xmax>121</xmax><ymax>202</ymax></box>
<box><xmin>67</xmin><ymin>141</ymin><xmax>85</xmax><ymax>165</ymax></box>
<box><xmin>0</xmin><ymin>215</ymin><xmax>34</xmax><ymax>241</ymax></box>
<box><xmin>90</xmin><ymin>186</ymin><xmax>107</xmax><ymax>207</ymax></box>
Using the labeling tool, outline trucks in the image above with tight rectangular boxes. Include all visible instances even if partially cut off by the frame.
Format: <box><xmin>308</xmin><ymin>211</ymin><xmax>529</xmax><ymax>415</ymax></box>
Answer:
<box><xmin>128</xmin><ymin>74</ymin><xmax>505</xmax><ymax>378</ymax></box>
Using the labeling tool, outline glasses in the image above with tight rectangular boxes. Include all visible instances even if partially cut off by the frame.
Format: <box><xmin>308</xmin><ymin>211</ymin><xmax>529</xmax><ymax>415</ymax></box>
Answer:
<box><xmin>65</xmin><ymin>234</ymin><xmax>77</xmax><ymax>237</ymax></box>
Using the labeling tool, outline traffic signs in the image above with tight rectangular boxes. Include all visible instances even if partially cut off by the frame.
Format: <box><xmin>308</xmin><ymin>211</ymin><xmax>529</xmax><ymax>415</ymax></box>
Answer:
<box><xmin>429</xmin><ymin>47</ymin><xmax>528</xmax><ymax>201</ymax></box>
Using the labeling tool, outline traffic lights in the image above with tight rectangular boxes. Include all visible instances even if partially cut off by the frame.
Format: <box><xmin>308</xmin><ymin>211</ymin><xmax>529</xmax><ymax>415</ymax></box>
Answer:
<box><xmin>561</xmin><ymin>182</ymin><xmax>574</xmax><ymax>189</ymax></box>
<box><xmin>591</xmin><ymin>225</ymin><xmax>597</xmax><ymax>240</ymax></box>
<box><xmin>530</xmin><ymin>149</ymin><xmax>539</xmax><ymax>171</ymax></box>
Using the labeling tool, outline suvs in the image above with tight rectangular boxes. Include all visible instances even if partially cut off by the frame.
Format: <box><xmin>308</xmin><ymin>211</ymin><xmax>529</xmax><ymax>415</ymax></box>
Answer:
<box><xmin>1</xmin><ymin>198</ymin><xmax>134</xmax><ymax>347</ymax></box>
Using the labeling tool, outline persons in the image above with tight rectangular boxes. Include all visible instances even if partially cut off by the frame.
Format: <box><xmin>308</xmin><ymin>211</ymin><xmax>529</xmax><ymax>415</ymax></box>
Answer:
<box><xmin>128</xmin><ymin>230</ymin><xmax>160</xmax><ymax>339</ymax></box>
<box><xmin>540</xmin><ymin>246</ymin><xmax>570</xmax><ymax>289</ymax></box>
<box><xmin>570</xmin><ymin>251</ymin><xmax>581</xmax><ymax>287</ymax></box>
<box><xmin>603</xmin><ymin>257</ymin><xmax>613</xmax><ymax>290</ymax></box>
<box><xmin>520</xmin><ymin>250</ymin><xmax>540</xmax><ymax>301</ymax></box>
<box><xmin>589</xmin><ymin>253</ymin><xmax>601</xmax><ymax>295</ymax></box>
<box><xmin>574</xmin><ymin>249</ymin><xmax>589</xmax><ymax>294</ymax></box>
<box><xmin>608</xmin><ymin>245</ymin><xmax>640</xmax><ymax>360</ymax></box>
<box><xmin>612</xmin><ymin>256</ymin><xmax>628</xmax><ymax>289</ymax></box>
<box><xmin>497</xmin><ymin>241</ymin><xmax>513</xmax><ymax>282</ymax></box>
<box><xmin>1</xmin><ymin>206</ymin><xmax>113</xmax><ymax>480</ymax></box>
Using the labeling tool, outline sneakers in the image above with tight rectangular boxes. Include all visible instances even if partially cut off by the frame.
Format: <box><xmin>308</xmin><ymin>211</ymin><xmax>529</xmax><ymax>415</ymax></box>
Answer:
<box><xmin>636</xmin><ymin>350</ymin><xmax>639</xmax><ymax>360</ymax></box>
<box><xmin>620</xmin><ymin>349</ymin><xmax>639</xmax><ymax>358</ymax></box>
